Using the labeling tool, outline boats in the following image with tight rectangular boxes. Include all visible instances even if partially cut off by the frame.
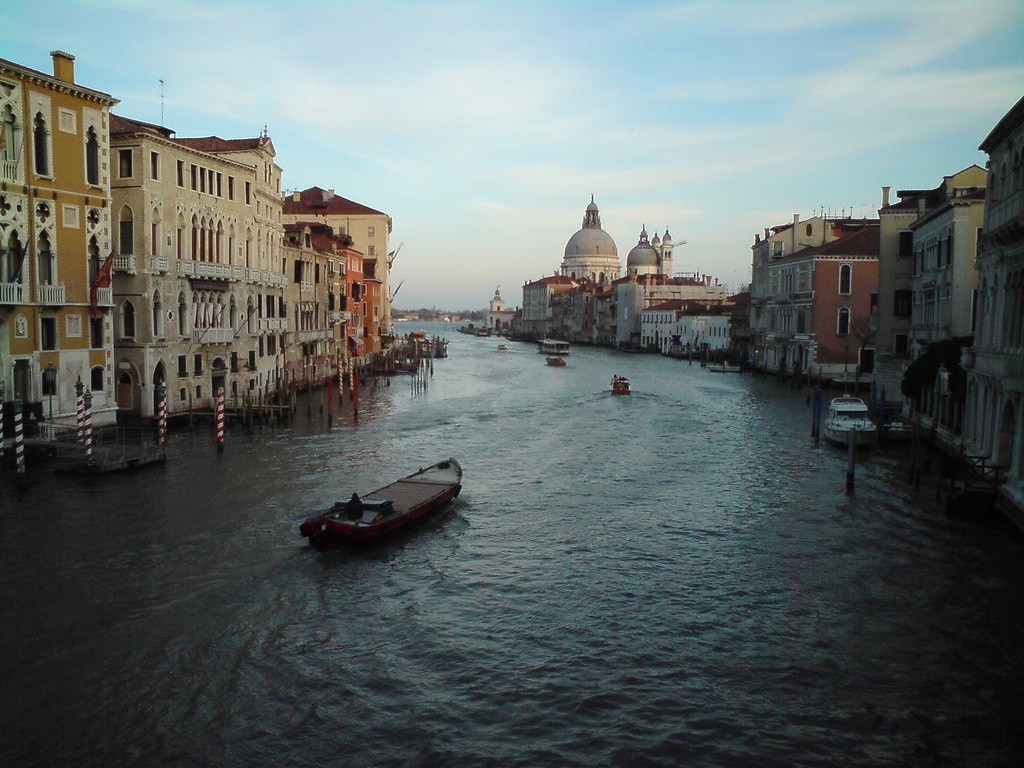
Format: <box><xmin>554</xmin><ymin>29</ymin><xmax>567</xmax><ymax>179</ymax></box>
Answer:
<box><xmin>707</xmin><ymin>361</ymin><xmax>740</xmax><ymax>372</ymax></box>
<box><xmin>832</xmin><ymin>371</ymin><xmax>873</xmax><ymax>391</ymax></box>
<box><xmin>612</xmin><ymin>377</ymin><xmax>630</xmax><ymax>394</ymax></box>
<box><xmin>372</xmin><ymin>338</ymin><xmax>446</xmax><ymax>372</ymax></box>
<box><xmin>824</xmin><ymin>393</ymin><xmax>879</xmax><ymax>445</ymax></box>
<box><xmin>867</xmin><ymin>398</ymin><xmax>917</xmax><ymax>439</ymax></box>
<box><xmin>546</xmin><ymin>357</ymin><xmax>566</xmax><ymax>366</ymax></box>
<box><xmin>536</xmin><ymin>339</ymin><xmax>570</xmax><ymax>354</ymax></box>
<box><xmin>300</xmin><ymin>457</ymin><xmax>462</xmax><ymax>545</ymax></box>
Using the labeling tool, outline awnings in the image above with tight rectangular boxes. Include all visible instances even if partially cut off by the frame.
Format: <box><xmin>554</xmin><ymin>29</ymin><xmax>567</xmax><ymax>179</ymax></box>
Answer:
<box><xmin>351</xmin><ymin>337</ymin><xmax>364</xmax><ymax>345</ymax></box>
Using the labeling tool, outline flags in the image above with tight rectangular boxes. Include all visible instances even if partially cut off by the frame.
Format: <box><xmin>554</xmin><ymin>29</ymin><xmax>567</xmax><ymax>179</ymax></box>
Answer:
<box><xmin>89</xmin><ymin>249</ymin><xmax>113</xmax><ymax>315</ymax></box>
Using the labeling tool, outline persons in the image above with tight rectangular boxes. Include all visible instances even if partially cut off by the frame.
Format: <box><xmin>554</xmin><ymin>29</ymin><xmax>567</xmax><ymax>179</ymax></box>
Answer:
<box><xmin>346</xmin><ymin>492</ymin><xmax>363</xmax><ymax>520</ymax></box>
<box><xmin>613</xmin><ymin>374</ymin><xmax>618</xmax><ymax>382</ymax></box>
